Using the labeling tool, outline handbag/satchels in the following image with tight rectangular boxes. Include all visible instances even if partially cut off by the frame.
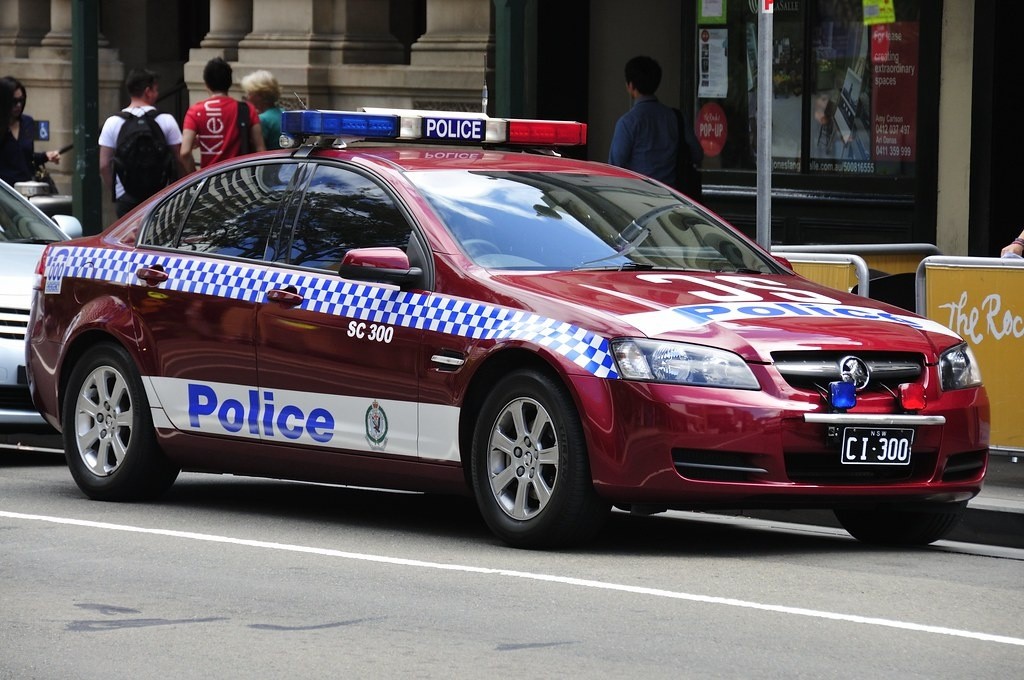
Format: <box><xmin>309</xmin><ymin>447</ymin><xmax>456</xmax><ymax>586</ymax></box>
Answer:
<box><xmin>32</xmin><ymin>159</ymin><xmax>59</xmax><ymax>194</ymax></box>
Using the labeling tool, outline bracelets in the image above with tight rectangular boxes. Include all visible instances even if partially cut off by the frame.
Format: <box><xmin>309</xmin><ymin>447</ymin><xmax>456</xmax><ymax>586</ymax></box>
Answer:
<box><xmin>1013</xmin><ymin>238</ymin><xmax>1024</xmax><ymax>248</ymax></box>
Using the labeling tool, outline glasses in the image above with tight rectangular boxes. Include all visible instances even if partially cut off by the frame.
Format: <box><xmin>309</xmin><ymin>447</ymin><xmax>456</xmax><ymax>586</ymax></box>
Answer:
<box><xmin>12</xmin><ymin>94</ymin><xmax>26</xmax><ymax>107</ymax></box>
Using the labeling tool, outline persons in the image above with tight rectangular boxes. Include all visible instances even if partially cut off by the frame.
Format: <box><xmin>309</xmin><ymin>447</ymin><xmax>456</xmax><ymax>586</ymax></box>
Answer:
<box><xmin>238</xmin><ymin>69</ymin><xmax>286</xmax><ymax>150</ymax></box>
<box><xmin>98</xmin><ymin>68</ymin><xmax>183</xmax><ymax>218</ymax></box>
<box><xmin>179</xmin><ymin>54</ymin><xmax>266</xmax><ymax>176</ymax></box>
<box><xmin>0</xmin><ymin>76</ymin><xmax>61</xmax><ymax>188</ymax></box>
<box><xmin>608</xmin><ymin>56</ymin><xmax>703</xmax><ymax>189</ymax></box>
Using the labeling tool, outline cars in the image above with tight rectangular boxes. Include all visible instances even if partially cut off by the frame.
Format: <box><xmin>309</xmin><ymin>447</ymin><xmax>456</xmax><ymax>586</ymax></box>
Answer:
<box><xmin>1</xmin><ymin>179</ymin><xmax>88</xmax><ymax>434</ymax></box>
<box><xmin>24</xmin><ymin>106</ymin><xmax>991</xmax><ymax>549</ymax></box>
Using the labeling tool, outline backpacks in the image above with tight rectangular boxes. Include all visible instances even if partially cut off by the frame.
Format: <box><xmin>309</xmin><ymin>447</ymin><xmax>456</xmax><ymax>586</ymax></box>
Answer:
<box><xmin>111</xmin><ymin>110</ymin><xmax>180</xmax><ymax>208</ymax></box>
